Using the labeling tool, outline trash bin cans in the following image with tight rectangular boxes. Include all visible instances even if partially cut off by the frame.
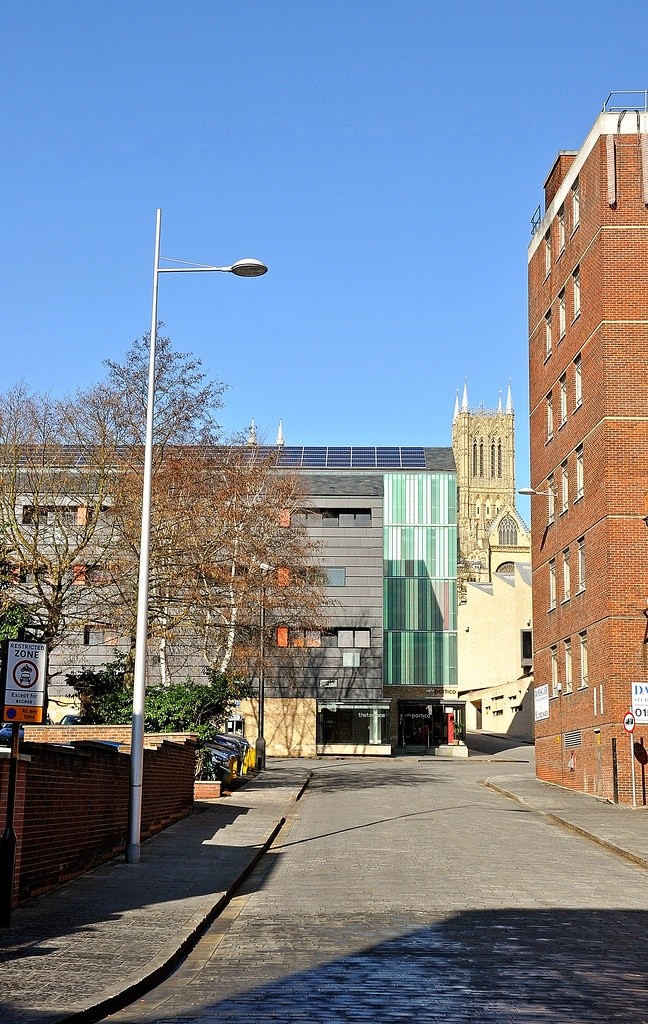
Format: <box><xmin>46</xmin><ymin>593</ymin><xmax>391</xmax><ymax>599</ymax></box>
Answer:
<box><xmin>228</xmin><ymin>715</ymin><xmax>245</xmax><ymax>737</ymax></box>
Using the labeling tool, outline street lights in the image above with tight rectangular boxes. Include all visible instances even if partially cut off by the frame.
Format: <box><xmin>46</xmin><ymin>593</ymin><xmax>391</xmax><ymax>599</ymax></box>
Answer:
<box><xmin>125</xmin><ymin>259</ymin><xmax>269</xmax><ymax>863</ymax></box>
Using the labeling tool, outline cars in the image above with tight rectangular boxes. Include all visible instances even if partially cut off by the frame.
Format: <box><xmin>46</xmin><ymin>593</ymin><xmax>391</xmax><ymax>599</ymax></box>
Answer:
<box><xmin>60</xmin><ymin>714</ymin><xmax>251</xmax><ymax>785</ymax></box>
<box><xmin>0</xmin><ymin>724</ymin><xmax>25</xmax><ymax>747</ymax></box>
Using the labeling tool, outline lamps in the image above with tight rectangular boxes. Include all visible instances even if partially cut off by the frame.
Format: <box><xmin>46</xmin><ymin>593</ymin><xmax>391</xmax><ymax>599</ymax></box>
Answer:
<box><xmin>517</xmin><ymin>485</ymin><xmax>558</xmax><ymax>499</ymax></box>
